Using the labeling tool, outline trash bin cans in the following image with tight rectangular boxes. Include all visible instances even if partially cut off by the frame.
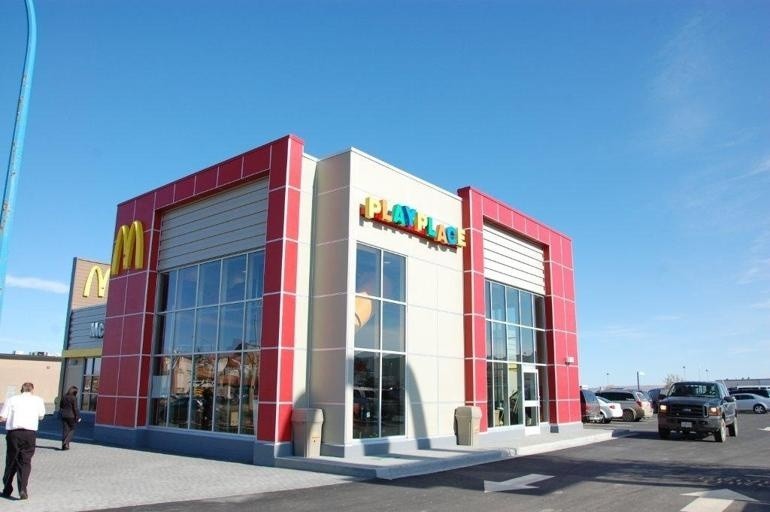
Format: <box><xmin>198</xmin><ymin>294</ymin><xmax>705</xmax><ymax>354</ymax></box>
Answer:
<box><xmin>456</xmin><ymin>405</ymin><xmax>482</xmax><ymax>446</ymax></box>
<box><xmin>291</xmin><ymin>408</ymin><xmax>324</xmax><ymax>456</ymax></box>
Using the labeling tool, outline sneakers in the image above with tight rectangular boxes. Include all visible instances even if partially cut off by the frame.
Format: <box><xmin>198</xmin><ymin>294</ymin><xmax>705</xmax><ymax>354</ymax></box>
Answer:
<box><xmin>20</xmin><ymin>491</ymin><xmax>28</xmax><ymax>499</ymax></box>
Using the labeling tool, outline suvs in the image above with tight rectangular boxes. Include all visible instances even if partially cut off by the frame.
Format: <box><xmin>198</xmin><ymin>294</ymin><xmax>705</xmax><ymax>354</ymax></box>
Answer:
<box><xmin>657</xmin><ymin>380</ymin><xmax>738</xmax><ymax>443</ymax></box>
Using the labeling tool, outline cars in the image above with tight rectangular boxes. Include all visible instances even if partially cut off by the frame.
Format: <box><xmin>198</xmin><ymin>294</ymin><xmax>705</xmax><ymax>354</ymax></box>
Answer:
<box><xmin>732</xmin><ymin>392</ymin><xmax>770</xmax><ymax>415</ymax></box>
<box><xmin>579</xmin><ymin>390</ymin><xmax>624</xmax><ymax>425</ymax></box>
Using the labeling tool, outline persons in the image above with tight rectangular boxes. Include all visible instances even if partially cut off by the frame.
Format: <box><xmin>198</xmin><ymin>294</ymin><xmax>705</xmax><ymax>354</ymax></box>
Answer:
<box><xmin>0</xmin><ymin>381</ymin><xmax>46</xmax><ymax>498</ymax></box>
<box><xmin>60</xmin><ymin>384</ymin><xmax>82</xmax><ymax>450</ymax></box>
<box><xmin>194</xmin><ymin>387</ymin><xmax>214</xmax><ymax>429</ymax></box>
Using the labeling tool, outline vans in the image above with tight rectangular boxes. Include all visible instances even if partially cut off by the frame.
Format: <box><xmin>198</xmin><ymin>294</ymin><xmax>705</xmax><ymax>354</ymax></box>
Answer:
<box><xmin>596</xmin><ymin>389</ymin><xmax>655</xmax><ymax>423</ymax></box>
